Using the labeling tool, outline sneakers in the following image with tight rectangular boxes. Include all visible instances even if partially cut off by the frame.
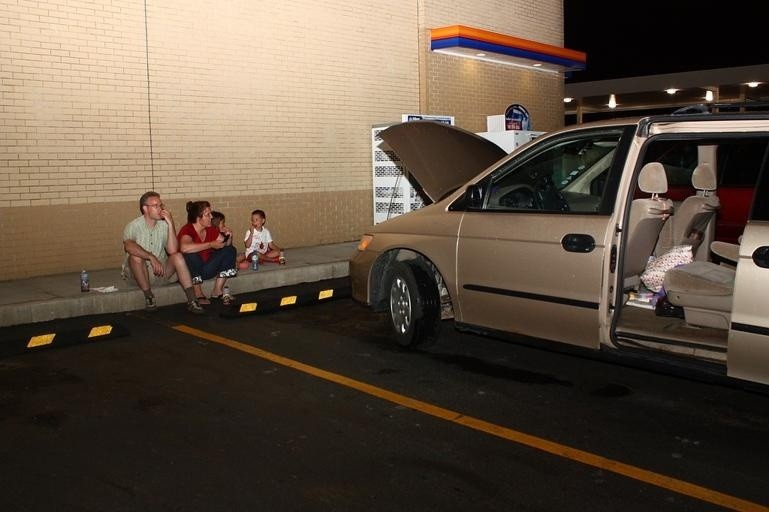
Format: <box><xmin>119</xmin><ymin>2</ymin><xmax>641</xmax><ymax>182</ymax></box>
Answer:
<box><xmin>144</xmin><ymin>293</ymin><xmax>157</xmax><ymax>313</ymax></box>
<box><xmin>185</xmin><ymin>299</ymin><xmax>205</xmax><ymax>315</ymax></box>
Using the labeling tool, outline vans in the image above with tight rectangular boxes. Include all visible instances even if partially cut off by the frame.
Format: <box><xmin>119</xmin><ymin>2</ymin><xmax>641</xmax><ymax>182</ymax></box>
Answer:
<box><xmin>349</xmin><ymin>111</ymin><xmax>768</xmax><ymax>390</ymax></box>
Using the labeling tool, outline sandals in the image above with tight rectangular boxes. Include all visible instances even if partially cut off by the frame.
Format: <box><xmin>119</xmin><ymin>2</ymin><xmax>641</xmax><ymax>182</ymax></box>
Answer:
<box><xmin>196</xmin><ymin>294</ymin><xmax>237</xmax><ymax>305</ymax></box>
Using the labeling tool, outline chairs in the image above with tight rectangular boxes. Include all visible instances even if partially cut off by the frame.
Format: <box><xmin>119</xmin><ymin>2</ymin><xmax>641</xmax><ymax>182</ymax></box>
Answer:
<box><xmin>622</xmin><ymin>162</ymin><xmax>742</xmax><ymax>332</ymax></box>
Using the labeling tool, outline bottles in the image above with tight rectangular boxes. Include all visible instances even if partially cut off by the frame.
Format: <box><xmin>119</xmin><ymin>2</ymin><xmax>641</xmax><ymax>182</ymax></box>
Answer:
<box><xmin>278</xmin><ymin>249</ymin><xmax>286</xmax><ymax>266</ymax></box>
<box><xmin>252</xmin><ymin>254</ymin><xmax>258</xmax><ymax>271</ymax></box>
<box><xmin>80</xmin><ymin>269</ymin><xmax>90</xmax><ymax>292</ymax></box>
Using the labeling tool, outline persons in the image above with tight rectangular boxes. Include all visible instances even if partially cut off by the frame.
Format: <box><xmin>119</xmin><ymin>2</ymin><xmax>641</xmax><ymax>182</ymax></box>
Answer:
<box><xmin>210</xmin><ymin>208</ymin><xmax>247</xmax><ymax>278</ymax></box>
<box><xmin>243</xmin><ymin>208</ymin><xmax>285</xmax><ymax>266</ymax></box>
<box><xmin>173</xmin><ymin>199</ymin><xmax>238</xmax><ymax>307</ymax></box>
<box><xmin>120</xmin><ymin>190</ymin><xmax>204</xmax><ymax>316</ymax></box>
